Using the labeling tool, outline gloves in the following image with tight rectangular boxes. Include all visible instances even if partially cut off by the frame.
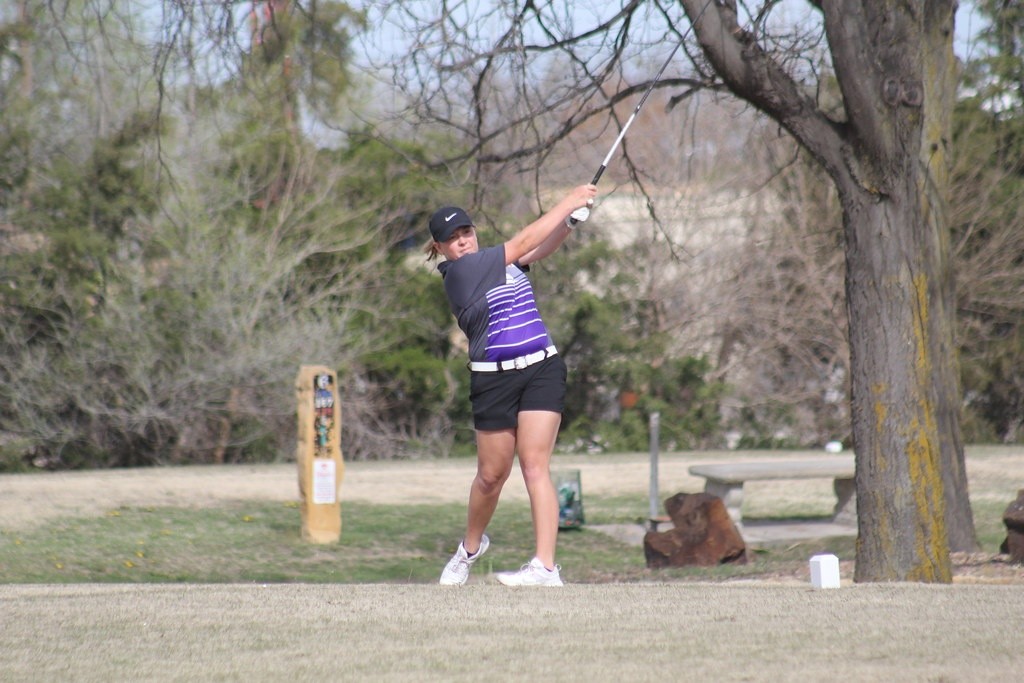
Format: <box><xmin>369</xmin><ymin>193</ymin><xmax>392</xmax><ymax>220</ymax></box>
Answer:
<box><xmin>564</xmin><ymin>207</ymin><xmax>590</xmax><ymax>229</ymax></box>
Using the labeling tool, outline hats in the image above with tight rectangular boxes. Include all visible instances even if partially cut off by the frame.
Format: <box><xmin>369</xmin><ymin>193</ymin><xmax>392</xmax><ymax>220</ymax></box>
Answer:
<box><xmin>429</xmin><ymin>207</ymin><xmax>476</xmax><ymax>242</ymax></box>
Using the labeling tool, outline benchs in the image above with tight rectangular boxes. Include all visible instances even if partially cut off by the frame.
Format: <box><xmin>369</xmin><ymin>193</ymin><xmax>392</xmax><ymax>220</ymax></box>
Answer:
<box><xmin>689</xmin><ymin>456</ymin><xmax>857</xmax><ymax>531</ymax></box>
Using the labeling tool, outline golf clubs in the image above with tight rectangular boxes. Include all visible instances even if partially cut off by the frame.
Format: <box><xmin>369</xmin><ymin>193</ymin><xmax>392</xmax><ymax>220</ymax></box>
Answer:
<box><xmin>568</xmin><ymin>0</ymin><xmax>713</xmax><ymax>223</ymax></box>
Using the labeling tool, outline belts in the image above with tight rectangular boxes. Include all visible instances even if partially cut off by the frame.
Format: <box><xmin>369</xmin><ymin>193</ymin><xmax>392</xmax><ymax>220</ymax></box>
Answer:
<box><xmin>471</xmin><ymin>345</ymin><xmax>559</xmax><ymax>372</ymax></box>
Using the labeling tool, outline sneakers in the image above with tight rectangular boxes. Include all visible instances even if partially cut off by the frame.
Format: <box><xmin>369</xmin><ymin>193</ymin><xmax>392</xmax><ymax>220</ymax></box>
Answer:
<box><xmin>496</xmin><ymin>558</ymin><xmax>565</xmax><ymax>587</ymax></box>
<box><xmin>439</xmin><ymin>533</ymin><xmax>489</xmax><ymax>585</ymax></box>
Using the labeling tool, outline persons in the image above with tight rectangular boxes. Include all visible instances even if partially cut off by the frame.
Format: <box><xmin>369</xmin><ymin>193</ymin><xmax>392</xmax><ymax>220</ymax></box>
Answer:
<box><xmin>427</xmin><ymin>184</ymin><xmax>596</xmax><ymax>587</ymax></box>
